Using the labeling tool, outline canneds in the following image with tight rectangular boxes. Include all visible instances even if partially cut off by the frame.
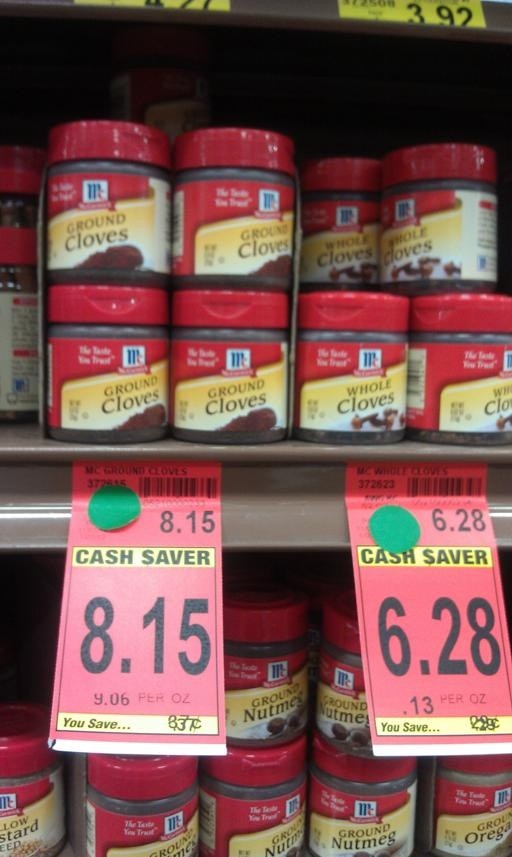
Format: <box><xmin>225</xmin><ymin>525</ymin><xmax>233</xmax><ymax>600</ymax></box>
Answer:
<box><xmin>1</xmin><ymin>46</ymin><xmax>511</xmax><ymax>446</ymax></box>
<box><xmin>1</xmin><ymin>550</ymin><xmax>512</xmax><ymax>857</ymax></box>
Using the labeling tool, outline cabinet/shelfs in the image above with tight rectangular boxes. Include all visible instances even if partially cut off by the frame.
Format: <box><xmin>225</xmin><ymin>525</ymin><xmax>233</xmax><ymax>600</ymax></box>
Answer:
<box><xmin>1</xmin><ymin>1</ymin><xmax>512</xmax><ymax>857</ymax></box>
